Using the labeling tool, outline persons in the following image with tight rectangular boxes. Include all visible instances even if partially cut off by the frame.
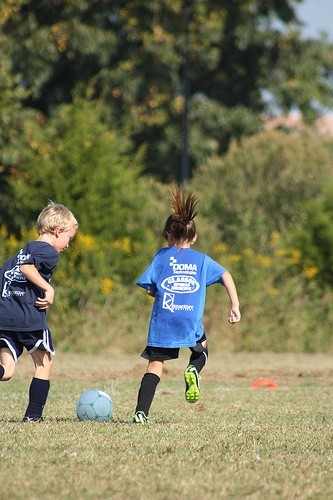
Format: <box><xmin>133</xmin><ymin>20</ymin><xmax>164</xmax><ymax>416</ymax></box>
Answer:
<box><xmin>130</xmin><ymin>212</ymin><xmax>241</xmax><ymax>424</ymax></box>
<box><xmin>0</xmin><ymin>202</ymin><xmax>80</xmax><ymax>422</ymax></box>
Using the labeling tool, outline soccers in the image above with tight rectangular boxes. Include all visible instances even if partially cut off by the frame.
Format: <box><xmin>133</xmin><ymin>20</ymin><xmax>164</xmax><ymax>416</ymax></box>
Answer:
<box><xmin>76</xmin><ymin>391</ymin><xmax>113</xmax><ymax>421</ymax></box>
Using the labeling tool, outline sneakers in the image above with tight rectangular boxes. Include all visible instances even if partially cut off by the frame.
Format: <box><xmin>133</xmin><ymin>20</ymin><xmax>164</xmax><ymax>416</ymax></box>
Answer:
<box><xmin>22</xmin><ymin>416</ymin><xmax>45</xmax><ymax>423</ymax></box>
<box><xmin>184</xmin><ymin>364</ymin><xmax>201</xmax><ymax>404</ymax></box>
<box><xmin>133</xmin><ymin>411</ymin><xmax>149</xmax><ymax>423</ymax></box>
